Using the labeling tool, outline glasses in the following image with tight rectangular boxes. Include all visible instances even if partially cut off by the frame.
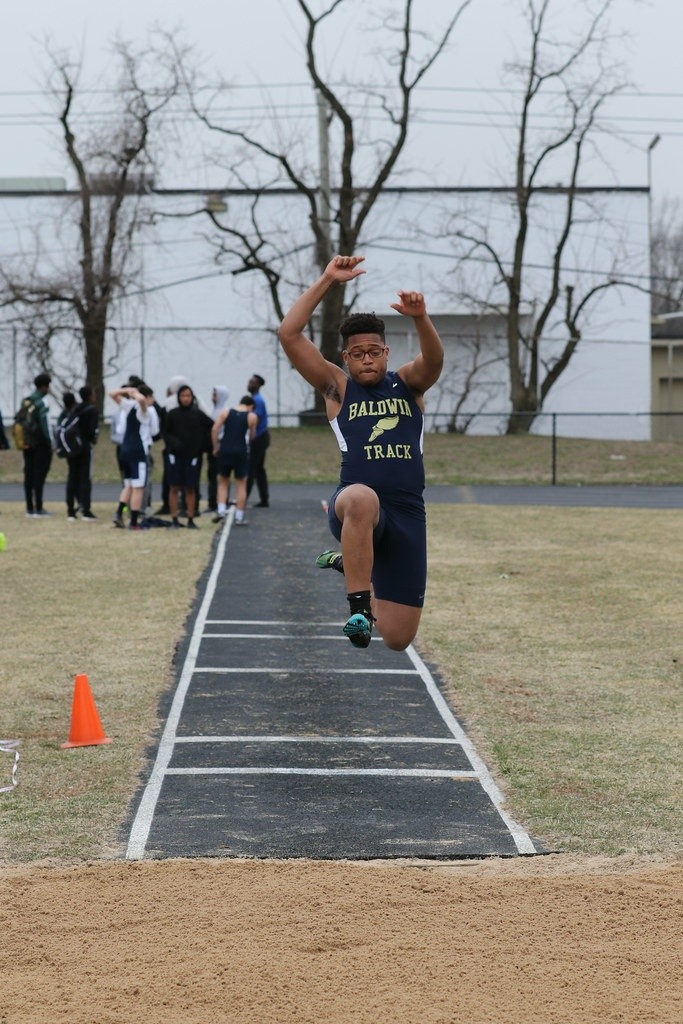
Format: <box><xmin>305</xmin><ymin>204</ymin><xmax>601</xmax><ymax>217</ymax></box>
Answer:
<box><xmin>346</xmin><ymin>346</ymin><xmax>387</xmax><ymax>361</ymax></box>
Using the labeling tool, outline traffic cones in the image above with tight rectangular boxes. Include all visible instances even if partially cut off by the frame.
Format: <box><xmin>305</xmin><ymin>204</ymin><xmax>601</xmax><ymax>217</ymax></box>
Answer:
<box><xmin>0</xmin><ymin>532</ymin><xmax>9</xmax><ymax>553</ymax></box>
<box><xmin>59</xmin><ymin>673</ymin><xmax>113</xmax><ymax>750</ymax></box>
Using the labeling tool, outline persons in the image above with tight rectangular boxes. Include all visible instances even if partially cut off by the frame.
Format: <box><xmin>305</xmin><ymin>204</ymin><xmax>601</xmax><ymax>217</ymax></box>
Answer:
<box><xmin>23</xmin><ymin>373</ymin><xmax>270</xmax><ymax>530</ymax></box>
<box><xmin>278</xmin><ymin>255</ymin><xmax>444</xmax><ymax>651</ymax></box>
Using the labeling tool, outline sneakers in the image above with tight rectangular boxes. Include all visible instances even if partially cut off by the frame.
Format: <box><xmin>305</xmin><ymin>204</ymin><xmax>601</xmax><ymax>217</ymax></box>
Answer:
<box><xmin>315</xmin><ymin>550</ymin><xmax>343</xmax><ymax>573</ymax></box>
<box><xmin>343</xmin><ymin>608</ymin><xmax>377</xmax><ymax>648</ymax></box>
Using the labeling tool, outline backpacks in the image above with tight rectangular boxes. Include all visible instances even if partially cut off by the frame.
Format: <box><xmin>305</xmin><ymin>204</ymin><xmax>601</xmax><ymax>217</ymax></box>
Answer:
<box><xmin>13</xmin><ymin>397</ymin><xmax>45</xmax><ymax>449</ymax></box>
<box><xmin>55</xmin><ymin>406</ymin><xmax>94</xmax><ymax>458</ymax></box>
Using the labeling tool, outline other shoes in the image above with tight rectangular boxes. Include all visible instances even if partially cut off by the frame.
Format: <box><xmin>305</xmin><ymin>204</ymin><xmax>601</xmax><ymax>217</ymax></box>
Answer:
<box><xmin>82</xmin><ymin>510</ymin><xmax>95</xmax><ymax>520</ymax></box>
<box><xmin>67</xmin><ymin>512</ymin><xmax>77</xmax><ymax>520</ymax></box>
<box><xmin>179</xmin><ymin>509</ymin><xmax>187</xmax><ymax>517</ymax></box>
<box><xmin>154</xmin><ymin>508</ymin><xmax>170</xmax><ymax>515</ymax></box>
<box><xmin>34</xmin><ymin>510</ymin><xmax>51</xmax><ymax>518</ymax></box>
<box><xmin>129</xmin><ymin>522</ymin><xmax>149</xmax><ymax>531</ymax></box>
<box><xmin>194</xmin><ymin>510</ymin><xmax>200</xmax><ymax>517</ymax></box>
<box><xmin>188</xmin><ymin>521</ymin><xmax>197</xmax><ymax>528</ymax></box>
<box><xmin>172</xmin><ymin>521</ymin><xmax>184</xmax><ymax>528</ymax></box>
<box><xmin>255</xmin><ymin>502</ymin><xmax>269</xmax><ymax>507</ymax></box>
<box><xmin>236</xmin><ymin>517</ymin><xmax>248</xmax><ymax>525</ymax></box>
<box><xmin>204</xmin><ymin>507</ymin><xmax>218</xmax><ymax>513</ymax></box>
<box><xmin>212</xmin><ymin>510</ymin><xmax>225</xmax><ymax>522</ymax></box>
<box><xmin>25</xmin><ymin>512</ymin><xmax>34</xmax><ymax>518</ymax></box>
<box><xmin>113</xmin><ymin>514</ymin><xmax>124</xmax><ymax>527</ymax></box>
